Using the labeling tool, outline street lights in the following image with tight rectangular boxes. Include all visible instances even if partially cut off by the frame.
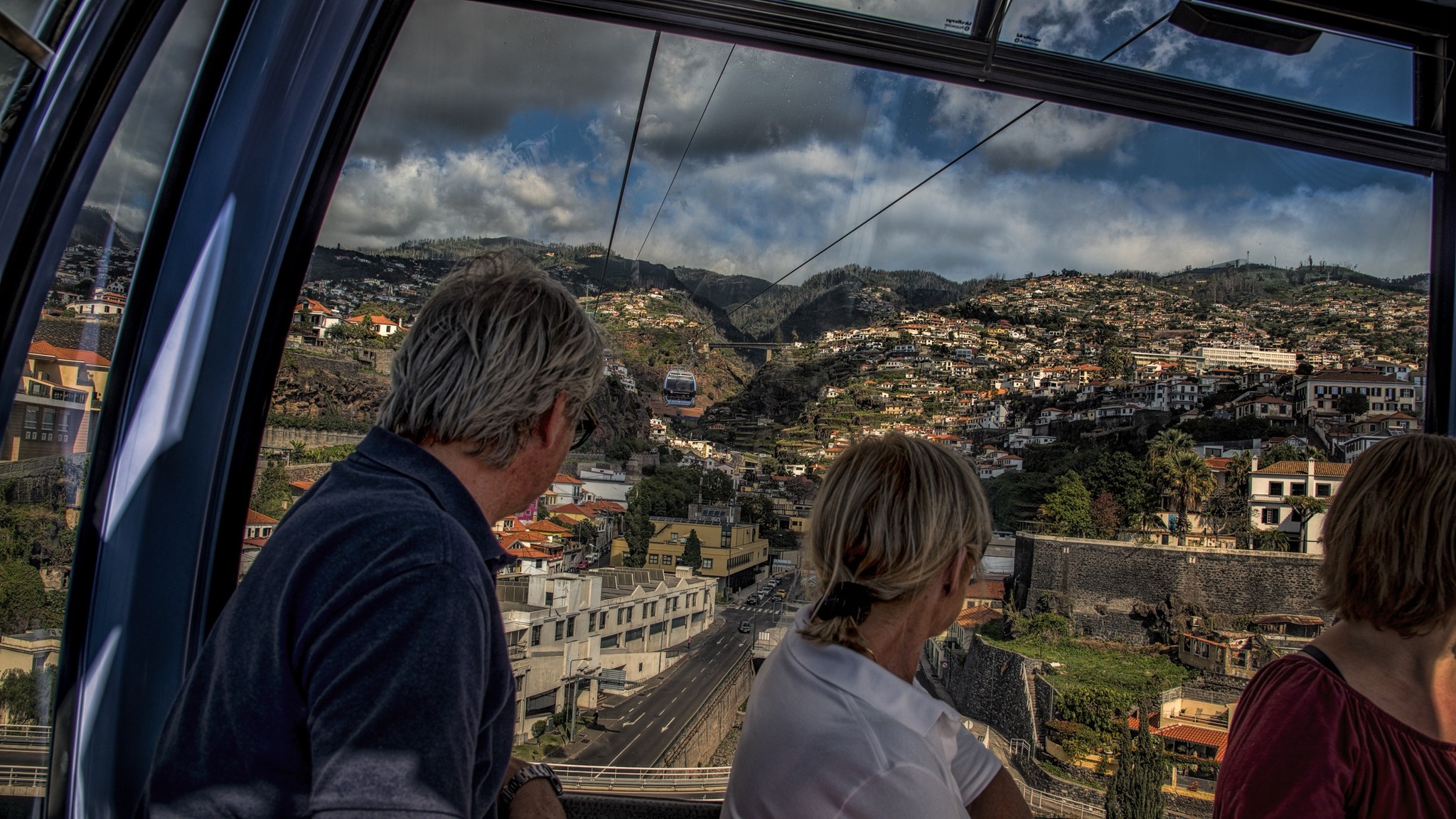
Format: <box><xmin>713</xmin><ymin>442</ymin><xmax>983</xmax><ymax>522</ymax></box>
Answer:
<box><xmin>752</xmin><ymin>580</ymin><xmax>763</xmax><ymax>650</ymax></box>
<box><xmin>589</xmin><ymin>543</ymin><xmax>600</xmax><ymax>569</ymax></box>
<box><xmin>701</xmin><ymin>577</ymin><xmax>720</xmax><ymax>633</ymax></box>
<box><xmin>769</xmin><ymin>534</ymin><xmax>783</xmax><ymax>576</ymax></box>
<box><xmin>658</xmin><ymin>606</ymin><xmax>681</xmax><ymax>674</ymax></box>
<box><xmin>565</xmin><ymin>657</ymin><xmax>592</xmax><ymax>747</ymax></box>
<box><xmin>728</xmin><ymin>548</ymin><xmax>744</xmax><ymax>592</ymax></box>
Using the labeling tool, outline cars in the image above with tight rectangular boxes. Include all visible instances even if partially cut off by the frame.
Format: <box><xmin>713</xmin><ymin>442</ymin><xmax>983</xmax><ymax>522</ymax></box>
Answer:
<box><xmin>776</xmin><ymin>589</ymin><xmax>787</xmax><ymax>598</ymax></box>
<box><xmin>768</xmin><ymin>580</ymin><xmax>777</xmax><ymax>588</ymax></box>
<box><xmin>747</xmin><ymin>594</ymin><xmax>761</xmax><ymax>605</ymax></box>
<box><xmin>772</xmin><ymin>594</ymin><xmax>783</xmax><ymax>603</ymax></box>
<box><xmin>568</xmin><ymin>531</ymin><xmax>627</xmax><ymax>574</ymax></box>
<box><xmin>765</xmin><ymin>584</ymin><xmax>775</xmax><ymax>592</ymax></box>
<box><xmin>738</xmin><ymin>621</ymin><xmax>752</xmax><ymax>633</ymax></box>
<box><xmin>761</xmin><ymin>587</ymin><xmax>771</xmax><ymax>596</ymax></box>
<box><xmin>757</xmin><ymin>591</ymin><xmax>764</xmax><ymax>600</ymax></box>
<box><xmin>773</xmin><ymin>577</ymin><xmax>783</xmax><ymax>585</ymax></box>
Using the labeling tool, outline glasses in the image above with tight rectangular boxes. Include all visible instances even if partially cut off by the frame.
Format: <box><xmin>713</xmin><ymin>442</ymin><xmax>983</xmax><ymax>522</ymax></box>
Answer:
<box><xmin>541</xmin><ymin>385</ymin><xmax>600</xmax><ymax>452</ymax></box>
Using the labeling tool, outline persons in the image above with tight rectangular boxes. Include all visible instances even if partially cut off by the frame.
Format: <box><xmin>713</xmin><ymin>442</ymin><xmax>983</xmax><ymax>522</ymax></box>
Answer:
<box><xmin>136</xmin><ymin>248</ymin><xmax>607</xmax><ymax>819</ymax></box>
<box><xmin>1212</xmin><ymin>432</ymin><xmax>1456</xmax><ymax>819</ymax></box>
<box><xmin>593</xmin><ymin>710</ymin><xmax>598</xmax><ymax>725</ymax></box>
<box><xmin>720</xmin><ymin>431</ymin><xmax>1033</xmax><ymax>819</ymax></box>
<box><xmin>687</xmin><ymin>636</ymin><xmax>692</xmax><ymax>649</ymax></box>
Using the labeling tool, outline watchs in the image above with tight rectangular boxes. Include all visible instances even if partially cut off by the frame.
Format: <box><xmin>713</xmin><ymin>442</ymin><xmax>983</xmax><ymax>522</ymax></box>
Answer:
<box><xmin>500</xmin><ymin>764</ymin><xmax>564</xmax><ymax>814</ymax></box>
